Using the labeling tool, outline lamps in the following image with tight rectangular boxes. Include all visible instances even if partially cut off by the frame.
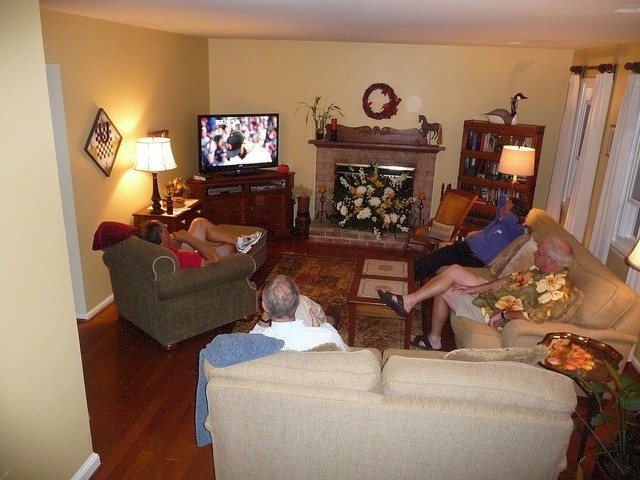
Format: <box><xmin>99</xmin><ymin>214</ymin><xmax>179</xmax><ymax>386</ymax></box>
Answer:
<box><xmin>498</xmin><ymin>146</ymin><xmax>536</xmax><ymax>208</ymax></box>
<box><xmin>624</xmin><ymin>237</ymin><xmax>640</xmax><ymax>272</ymax></box>
<box><xmin>133</xmin><ymin>137</ymin><xmax>178</xmax><ymax>214</ymax></box>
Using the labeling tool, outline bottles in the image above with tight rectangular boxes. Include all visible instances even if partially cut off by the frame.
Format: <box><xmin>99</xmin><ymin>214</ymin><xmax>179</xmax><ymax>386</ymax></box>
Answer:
<box><xmin>167</xmin><ymin>190</ymin><xmax>173</xmax><ymax>214</ymax></box>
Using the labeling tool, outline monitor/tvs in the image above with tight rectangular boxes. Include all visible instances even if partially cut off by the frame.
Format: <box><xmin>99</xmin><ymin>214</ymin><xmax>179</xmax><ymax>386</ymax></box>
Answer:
<box><xmin>197</xmin><ymin>113</ymin><xmax>279</xmax><ymax>176</ymax></box>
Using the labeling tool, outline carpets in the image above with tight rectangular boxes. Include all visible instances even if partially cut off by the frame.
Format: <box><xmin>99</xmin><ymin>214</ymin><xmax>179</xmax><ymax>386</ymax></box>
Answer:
<box><xmin>229</xmin><ymin>251</ymin><xmax>428</xmax><ymax>350</ymax></box>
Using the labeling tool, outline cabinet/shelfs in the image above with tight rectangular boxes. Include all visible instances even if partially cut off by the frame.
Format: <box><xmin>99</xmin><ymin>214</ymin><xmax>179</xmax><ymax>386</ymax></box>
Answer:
<box><xmin>185</xmin><ymin>169</ymin><xmax>295</xmax><ymax>238</ymax></box>
<box><xmin>457</xmin><ymin>119</ymin><xmax>545</xmax><ymax>215</ymax></box>
<box><xmin>132</xmin><ymin>196</ymin><xmax>202</xmax><ymax>235</ymax></box>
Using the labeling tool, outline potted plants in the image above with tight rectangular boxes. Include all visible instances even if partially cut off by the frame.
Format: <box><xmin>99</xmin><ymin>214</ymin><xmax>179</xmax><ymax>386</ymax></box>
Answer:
<box><xmin>165</xmin><ymin>177</ymin><xmax>191</xmax><ymax>197</ymax></box>
<box><xmin>291</xmin><ymin>184</ymin><xmax>312</xmax><ymax>212</ymax></box>
<box><xmin>292</xmin><ymin>93</ymin><xmax>346</xmax><ymax>140</ymax></box>
<box><xmin>564</xmin><ymin>354</ymin><xmax>640</xmax><ymax>480</ymax></box>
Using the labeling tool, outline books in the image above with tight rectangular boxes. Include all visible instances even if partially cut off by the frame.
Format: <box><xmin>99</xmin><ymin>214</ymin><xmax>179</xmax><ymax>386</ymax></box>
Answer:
<box><xmin>461</xmin><ymin>183</ymin><xmax>526</xmax><ymax>208</ymax></box>
<box><xmin>464</xmin><ymin>157</ymin><xmax>526</xmax><ymax>184</ymax></box>
<box><xmin>468</xmin><ymin>131</ymin><xmax>533</xmax><ymax>155</ymax></box>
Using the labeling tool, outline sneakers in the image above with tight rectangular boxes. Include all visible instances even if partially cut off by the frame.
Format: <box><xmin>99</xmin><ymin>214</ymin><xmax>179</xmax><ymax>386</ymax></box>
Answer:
<box><xmin>234</xmin><ymin>230</ymin><xmax>263</xmax><ymax>255</ymax></box>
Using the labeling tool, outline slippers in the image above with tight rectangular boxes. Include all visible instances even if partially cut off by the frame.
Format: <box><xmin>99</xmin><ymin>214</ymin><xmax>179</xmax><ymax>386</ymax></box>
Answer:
<box><xmin>376</xmin><ymin>288</ymin><xmax>410</xmax><ymax>319</ymax></box>
<box><xmin>409</xmin><ymin>334</ymin><xmax>443</xmax><ymax>352</ymax></box>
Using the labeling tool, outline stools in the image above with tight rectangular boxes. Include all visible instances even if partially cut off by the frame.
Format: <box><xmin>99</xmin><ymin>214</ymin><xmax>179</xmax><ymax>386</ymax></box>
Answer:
<box><xmin>205</xmin><ymin>222</ymin><xmax>268</xmax><ymax>272</ymax></box>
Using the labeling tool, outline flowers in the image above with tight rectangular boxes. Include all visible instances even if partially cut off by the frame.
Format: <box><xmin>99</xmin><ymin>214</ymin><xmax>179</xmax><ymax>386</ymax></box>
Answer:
<box><xmin>511</xmin><ymin>270</ymin><xmax>534</xmax><ymax>290</ymax></box>
<box><xmin>495</xmin><ymin>295</ymin><xmax>522</xmax><ymax>311</ymax></box>
<box><xmin>536</xmin><ymin>274</ymin><xmax>564</xmax><ymax>303</ymax></box>
<box><xmin>482</xmin><ymin>307</ymin><xmax>493</xmax><ymax>322</ymax></box>
<box><xmin>327</xmin><ymin>160</ymin><xmax>418</xmax><ymax>240</ymax></box>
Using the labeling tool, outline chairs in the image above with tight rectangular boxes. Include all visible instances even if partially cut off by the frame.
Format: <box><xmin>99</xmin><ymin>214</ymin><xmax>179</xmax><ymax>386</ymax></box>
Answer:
<box><xmin>401</xmin><ymin>183</ymin><xmax>478</xmax><ymax>259</ymax></box>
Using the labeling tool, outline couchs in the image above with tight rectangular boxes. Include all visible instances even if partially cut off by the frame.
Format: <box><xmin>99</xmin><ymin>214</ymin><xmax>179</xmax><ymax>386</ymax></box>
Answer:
<box><xmin>203</xmin><ymin>345</ymin><xmax>578</xmax><ymax>480</ymax></box>
<box><xmin>446</xmin><ymin>208</ymin><xmax>640</xmax><ymax>355</ymax></box>
<box><xmin>99</xmin><ymin>236</ymin><xmax>258</xmax><ymax>351</ymax></box>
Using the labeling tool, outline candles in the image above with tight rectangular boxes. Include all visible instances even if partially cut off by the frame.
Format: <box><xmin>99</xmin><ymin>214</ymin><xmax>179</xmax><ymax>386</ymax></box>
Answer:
<box><xmin>320</xmin><ymin>184</ymin><xmax>327</xmax><ymax>192</ymax></box>
<box><xmin>330</xmin><ymin>118</ymin><xmax>337</xmax><ymax>130</ymax></box>
<box><xmin>412</xmin><ymin>192</ymin><xmax>425</xmax><ymax>228</ymax></box>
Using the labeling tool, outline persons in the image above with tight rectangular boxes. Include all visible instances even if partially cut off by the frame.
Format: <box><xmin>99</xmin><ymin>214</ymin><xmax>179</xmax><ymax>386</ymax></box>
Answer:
<box><xmin>247</xmin><ymin>273</ymin><xmax>340</xmax><ymax>352</ymax></box>
<box><xmin>415</xmin><ymin>194</ymin><xmax>531</xmax><ymax>282</ymax></box>
<box><xmin>201</xmin><ymin>117</ymin><xmax>278</xmax><ymax>168</ymax></box>
<box><xmin>377</xmin><ymin>237</ymin><xmax>575</xmax><ymax>352</ymax></box>
<box><xmin>140</xmin><ymin>218</ymin><xmax>263</xmax><ymax>271</ymax></box>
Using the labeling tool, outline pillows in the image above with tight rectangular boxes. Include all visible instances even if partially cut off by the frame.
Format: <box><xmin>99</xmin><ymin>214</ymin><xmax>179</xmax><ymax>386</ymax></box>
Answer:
<box><xmin>444</xmin><ymin>348</ymin><xmax>547</xmax><ymax>366</ymax></box>
<box><xmin>428</xmin><ymin>218</ymin><xmax>454</xmax><ymax>241</ymax></box>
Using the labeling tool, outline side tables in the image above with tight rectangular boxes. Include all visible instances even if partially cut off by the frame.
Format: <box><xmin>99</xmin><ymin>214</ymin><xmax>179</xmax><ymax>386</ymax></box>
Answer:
<box><xmin>533</xmin><ymin>331</ymin><xmax>623</xmax><ymax>465</ymax></box>
